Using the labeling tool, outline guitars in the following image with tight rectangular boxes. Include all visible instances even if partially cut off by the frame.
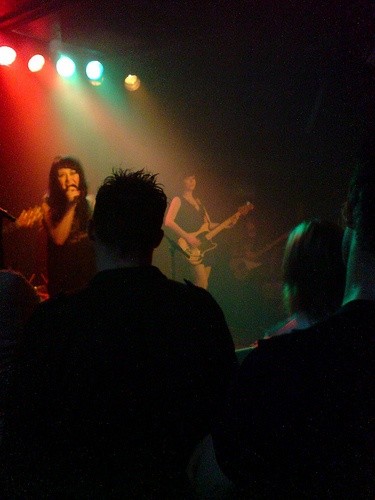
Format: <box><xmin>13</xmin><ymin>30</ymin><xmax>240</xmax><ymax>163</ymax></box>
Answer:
<box><xmin>178</xmin><ymin>202</ymin><xmax>255</xmax><ymax>267</ymax></box>
<box><xmin>227</xmin><ymin>233</ymin><xmax>289</xmax><ymax>283</ymax></box>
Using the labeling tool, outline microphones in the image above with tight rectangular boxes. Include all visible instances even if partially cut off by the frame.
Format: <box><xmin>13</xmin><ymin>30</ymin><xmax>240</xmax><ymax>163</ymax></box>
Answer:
<box><xmin>69</xmin><ymin>184</ymin><xmax>81</xmax><ymax>205</ymax></box>
<box><xmin>0</xmin><ymin>207</ymin><xmax>16</xmax><ymax>222</ymax></box>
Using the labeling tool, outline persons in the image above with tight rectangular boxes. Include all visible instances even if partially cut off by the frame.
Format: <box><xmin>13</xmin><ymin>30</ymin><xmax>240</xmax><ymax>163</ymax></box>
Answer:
<box><xmin>1</xmin><ymin>154</ymin><xmax>375</xmax><ymax>500</ymax></box>
